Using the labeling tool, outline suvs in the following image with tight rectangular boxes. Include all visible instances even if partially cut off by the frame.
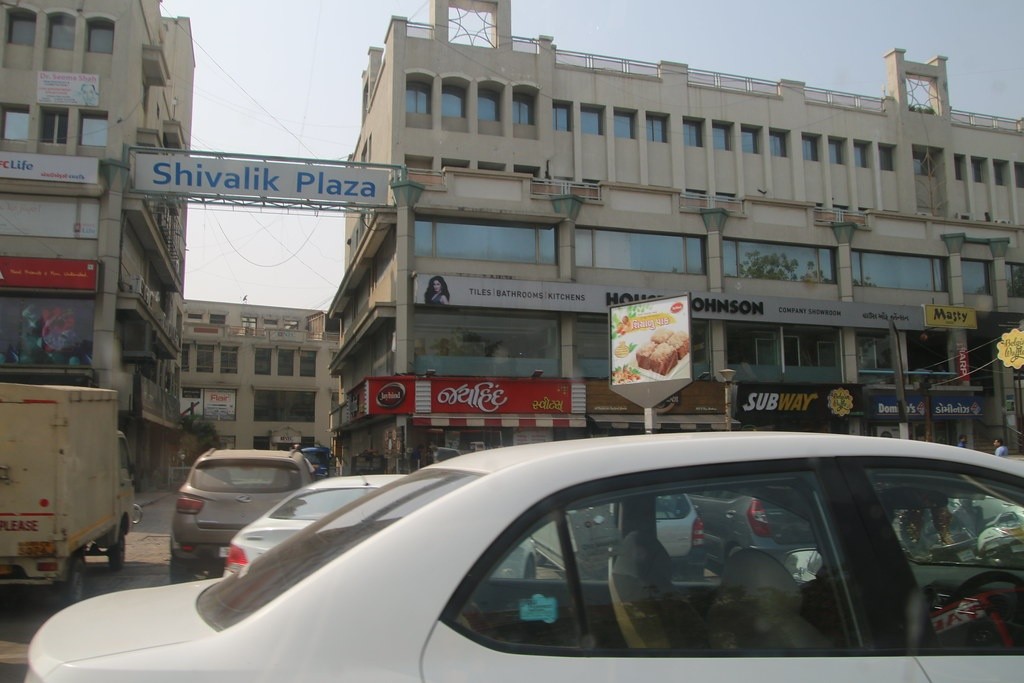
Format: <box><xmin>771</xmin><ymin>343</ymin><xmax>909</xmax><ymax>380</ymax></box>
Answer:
<box><xmin>170</xmin><ymin>445</ymin><xmax>315</xmax><ymax>565</ymax></box>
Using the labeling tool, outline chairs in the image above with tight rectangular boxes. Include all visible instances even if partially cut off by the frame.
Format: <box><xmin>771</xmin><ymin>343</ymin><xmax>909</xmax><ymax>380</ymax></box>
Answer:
<box><xmin>706</xmin><ymin>548</ymin><xmax>833</xmax><ymax>649</ymax></box>
<box><xmin>609</xmin><ymin>530</ymin><xmax>699</xmax><ymax>649</ymax></box>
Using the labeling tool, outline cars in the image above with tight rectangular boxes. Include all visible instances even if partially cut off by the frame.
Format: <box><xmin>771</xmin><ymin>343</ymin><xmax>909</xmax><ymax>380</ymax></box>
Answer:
<box><xmin>655</xmin><ymin>493</ymin><xmax>707</xmax><ymax>566</ymax></box>
<box><xmin>24</xmin><ymin>431</ymin><xmax>1024</xmax><ymax>683</ymax></box>
<box><xmin>947</xmin><ymin>493</ymin><xmax>1024</xmax><ymax>520</ymax></box>
<box><xmin>297</xmin><ymin>446</ymin><xmax>329</xmax><ymax>477</ymax></box>
<box><xmin>689</xmin><ymin>491</ymin><xmax>819</xmax><ymax>576</ymax></box>
<box><xmin>222</xmin><ymin>473</ymin><xmax>407</xmax><ymax>576</ymax></box>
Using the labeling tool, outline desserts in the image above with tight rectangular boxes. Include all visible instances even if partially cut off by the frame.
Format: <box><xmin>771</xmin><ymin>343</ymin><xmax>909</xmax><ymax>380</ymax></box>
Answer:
<box><xmin>635</xmin><ymin>327</ymin><xmax>689</xmax><ymax>376</ymax></box>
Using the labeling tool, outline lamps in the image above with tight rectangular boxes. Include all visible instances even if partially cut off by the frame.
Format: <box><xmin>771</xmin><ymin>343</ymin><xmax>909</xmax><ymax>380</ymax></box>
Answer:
<box><xmin>425</xmin><ymin>370</ymin><xmax>436</xmax><ymax>376</ymax></box>
<box><xmin>532</xmin><ymin>370</ymin><xmax>543</xmax><ymax>377</ymax></box>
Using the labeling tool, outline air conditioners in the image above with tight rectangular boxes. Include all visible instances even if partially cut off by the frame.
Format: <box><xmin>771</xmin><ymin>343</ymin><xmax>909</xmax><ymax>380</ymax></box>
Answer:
<box><xmin>132</xmin><ymin>275</ymin><xmax>155</xmax><ymax>311</ymax></box>
<box><xmin>166</xmin><ymin>323</ymin><xmax>182</xmax><ymax>351</ymax></box>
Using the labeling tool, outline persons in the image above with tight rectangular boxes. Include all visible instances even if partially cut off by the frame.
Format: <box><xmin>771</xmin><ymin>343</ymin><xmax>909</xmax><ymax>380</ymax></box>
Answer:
<box><xmin>424</xmin><ymin>275</ymin><xmax>450</xmax><ymax>305</ymax></box>
<box><xmin>800</xmin><ymin>543</ymin><xmax>859</xmax><ymax>649</ymax></box>
<box><xmin>959</xmin><ymin>434</ymin><xmax>969</xmax><ymax>448</ymax></box>
<box><xmin>994</xmin><ymin>439</ymin><xmax>1008</xmax><ymax>458</ymax></box>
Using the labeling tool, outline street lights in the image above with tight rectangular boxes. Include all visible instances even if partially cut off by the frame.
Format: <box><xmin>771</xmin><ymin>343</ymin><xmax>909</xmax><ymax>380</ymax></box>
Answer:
<box><xmin>878</xmin><ymin>315</ymin><xmax>908</xmax><ymax>439</ymax></box>
<box><xmin>719</xmin><ymin>368</ymin><xmax>736</xmax><ymax>431</ymax></box>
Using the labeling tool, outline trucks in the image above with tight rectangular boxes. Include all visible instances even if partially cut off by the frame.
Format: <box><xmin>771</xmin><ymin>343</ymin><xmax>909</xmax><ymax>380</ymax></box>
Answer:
<box><xmin>531</xmin><ymin>498</ymin><xmax>630</xmax><ymax>577</ymax></box>
<box><xmin>0</xmin><ymin>378</ymin><xmax>145</xmax><ymax>610</ymax></box>
